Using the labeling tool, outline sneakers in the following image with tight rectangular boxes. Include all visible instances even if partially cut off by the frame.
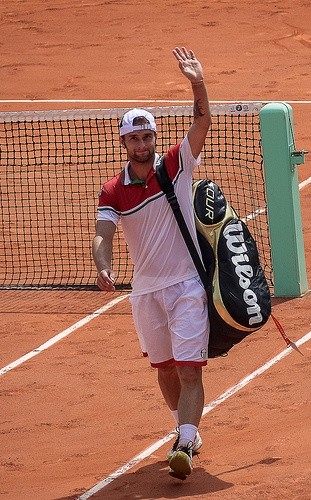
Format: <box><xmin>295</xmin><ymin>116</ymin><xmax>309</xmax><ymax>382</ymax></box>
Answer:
<box><xmin>167</xmin><ymin>431</ymin><xmax>202</xmax><ymax>461</ymax></box>
<box><xmin>168</xmin><ymin>449</ymin><xmax>193</xmax><ymax>481</ymax></box>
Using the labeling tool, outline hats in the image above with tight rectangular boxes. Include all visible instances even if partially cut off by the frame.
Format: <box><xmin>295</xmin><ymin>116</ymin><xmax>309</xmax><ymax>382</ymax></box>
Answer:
<box><xmin>120</xmin><ymin>109</ymin><xmax>158</xmax><ymax>136</ymax></box>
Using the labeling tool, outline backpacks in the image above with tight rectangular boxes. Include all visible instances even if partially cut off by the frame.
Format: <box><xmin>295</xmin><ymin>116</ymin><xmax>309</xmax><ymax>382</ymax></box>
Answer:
<box><xmin>191</xmin><ymin>177</ymin><xmax>271</xmax><ymax>359</ymax></box>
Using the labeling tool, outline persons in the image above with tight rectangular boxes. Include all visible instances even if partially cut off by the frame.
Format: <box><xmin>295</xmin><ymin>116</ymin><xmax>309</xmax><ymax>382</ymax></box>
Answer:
<box><xmin>91</xmin><ymin>47</ymin><xmax>214</xmax><ymax>482</ymax></box>
